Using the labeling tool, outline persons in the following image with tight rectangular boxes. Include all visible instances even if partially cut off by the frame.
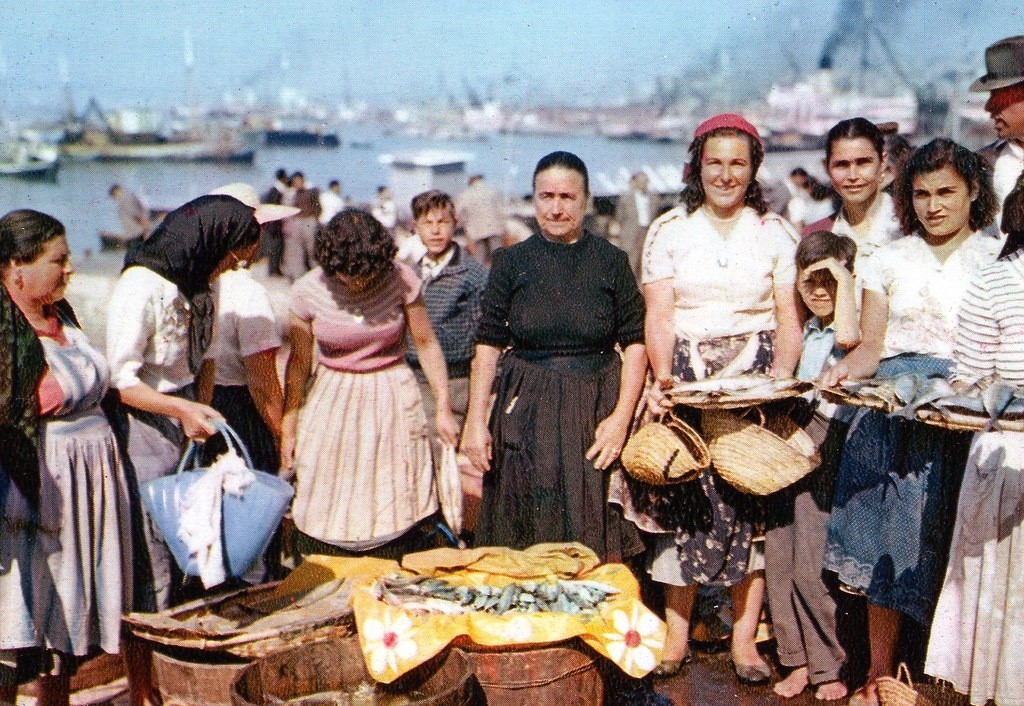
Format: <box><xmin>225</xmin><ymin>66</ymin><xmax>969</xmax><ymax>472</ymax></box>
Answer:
<box><xmin>106</xmin><ymin>195</ymin><xmax>260</xmax><ymax>706</ymax></box>
<box><xmin>263</xmin><ymin>170</ymin><xmax>345</xmax><ymax>278</ymax></box>
<box><xmin>457</xmin><ymin>175</ymin><xmax>502</xmax><ymax>264</ymax></box>
<box><xmin>766</xmin><ymin>167</ymin><xmax>834</xmax><ymax>238</ymax></box>
<box><xmin>209</xmin><ymin>181</ymin><xmax>301</xmax><ymax>474</ymax></box>
<box><xmin>370</xmin><ymin>187</ymin><xmax>396</xmax><ymax>239</ymax></box>
<box><xmin>460</xmin><ymin>152</ymin><xmax>649</xmax><ymax>562</ymax></box>
<box><xmin>974</xmin><ymin>32</ymin><xmax>1023</xmax><ymax>211</ymax></box>
<box><xmin>614</xmin><ymin>172</ymin><xmax>658</xmax><ymax>283</ymax></box>
<box><xmin>109</xmin><ymin>184</ymin><xmax>147</xmax><ymax>247</ymax></box>
<box><xmin>401</xmin><ymin>190</ymin><xmax>488</xmax><ymax>475</ymax></box>
<box><xmin>766</xmin><ymin>230</ymin><xmax>861</xmax><ymax>702</ymax></box>
<box><xmin>0</xmin><ymin>208</ymin><xmax>132</xmax><ymax>706</ymax></box>
<box><xmin>820</xmin><ymin>118</ymin><xmax>1001</xmax><ymax>704</ymax></box>
<box><xmin>641</xmin><ymin>112</ymin><xmax>805</xmax><ymax>683</ymax></box>
<box><xmin>282</xmin><ymin>209</ymin><xmax>460</xmax><ymax>557</ymax></box>
<box><xmin>924</xmin><ymin>170</ymin><xmax>1024</xmax><ymax>706</ymax></box>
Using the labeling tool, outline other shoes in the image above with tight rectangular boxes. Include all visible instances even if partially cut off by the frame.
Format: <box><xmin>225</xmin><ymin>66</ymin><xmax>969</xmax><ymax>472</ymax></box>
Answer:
<box><xmin>654</xmin><ymin>651</ymin><xmax>694</xmax><ymax>677</ymax></box>
<box><xmin>728</xmin><ymin>645</ymin><xmax>772</xmax><ymax>682</ymax></box>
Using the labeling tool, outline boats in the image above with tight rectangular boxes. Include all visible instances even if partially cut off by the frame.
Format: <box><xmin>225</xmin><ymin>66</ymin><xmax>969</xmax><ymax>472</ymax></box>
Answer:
<box><xmin>0</xmin><ymin>3</ymin><xmax>926</xmax><ymax>190</ymax></box>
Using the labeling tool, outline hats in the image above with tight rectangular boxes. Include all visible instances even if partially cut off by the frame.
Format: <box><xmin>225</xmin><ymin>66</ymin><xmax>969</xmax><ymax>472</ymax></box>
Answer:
<box><xmin>970</xmin><ymin>36</ymin><xmax>1024</xmax><ymax>92</ymax></box>
<box><xmin>210</xmin><ymin>182</ymin><xmax>301</xmax><ymax>224</ymax></box>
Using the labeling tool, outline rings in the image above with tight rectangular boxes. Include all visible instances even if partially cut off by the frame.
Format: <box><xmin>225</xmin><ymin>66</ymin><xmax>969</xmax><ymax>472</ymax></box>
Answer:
<box><xmin>612</xmin><ymin>449</ymin><xmax>618</xmax><ymax>455</ymax></box>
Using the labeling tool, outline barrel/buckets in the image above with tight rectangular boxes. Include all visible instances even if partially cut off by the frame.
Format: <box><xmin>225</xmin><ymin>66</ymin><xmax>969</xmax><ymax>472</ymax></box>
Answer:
<box><xmin>229</xmin><ymin>636</ymin><xmax>475</xmax><ymax>706</ymax></box>
<box><xmin>117</xmin><ymin>624</ymin><xmax>268</xmax><ymax>706</ymax></box>
<box><xmin>458</xmin><ymin>639</ymin><xmax>607</xmax><ymax>705</ymax></box>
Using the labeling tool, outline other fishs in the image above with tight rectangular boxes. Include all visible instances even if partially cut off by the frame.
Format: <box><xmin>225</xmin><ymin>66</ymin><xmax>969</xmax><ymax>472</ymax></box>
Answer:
<box><xmin>391</xmin><ymin>576</ymin><xmax>622</xmax><ymax>616</ymax></box>
<box><xmin>840</xmin><ymin>370</ymin><xmax>1024</xmax><ymax>434</ymax></box>
<box><xmin>664</xmin><ymin>374</ymin><xmax>802</xmax><ymax>399</ymax></box>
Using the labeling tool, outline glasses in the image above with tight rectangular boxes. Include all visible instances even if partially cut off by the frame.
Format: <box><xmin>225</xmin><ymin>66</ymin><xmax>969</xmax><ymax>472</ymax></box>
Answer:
<box><xmin>227</xmin><ymin>250</ymin><xmax>248</xmax><ymax>269</ymax></box>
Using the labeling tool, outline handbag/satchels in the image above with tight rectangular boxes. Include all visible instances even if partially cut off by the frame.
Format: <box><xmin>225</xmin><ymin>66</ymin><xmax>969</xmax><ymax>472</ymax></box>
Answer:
<box><xmin>876</xmin><ymin>662</ymin><xmax>993</xmax><ymax>706</ymax></box>
<box><xmin>620</xmin><ymin>404</ymin><xmax>710</xmax><ymax>486</ymax></box>
<box><xmin>701</xmin><ymin>403</ymin><xmax>821</xmax><ymax>495</ymax></box>
<box><xmin>140</xmin><ymin>420</ymin><xmax>294</xmax><ymax>576</ymax></box>
